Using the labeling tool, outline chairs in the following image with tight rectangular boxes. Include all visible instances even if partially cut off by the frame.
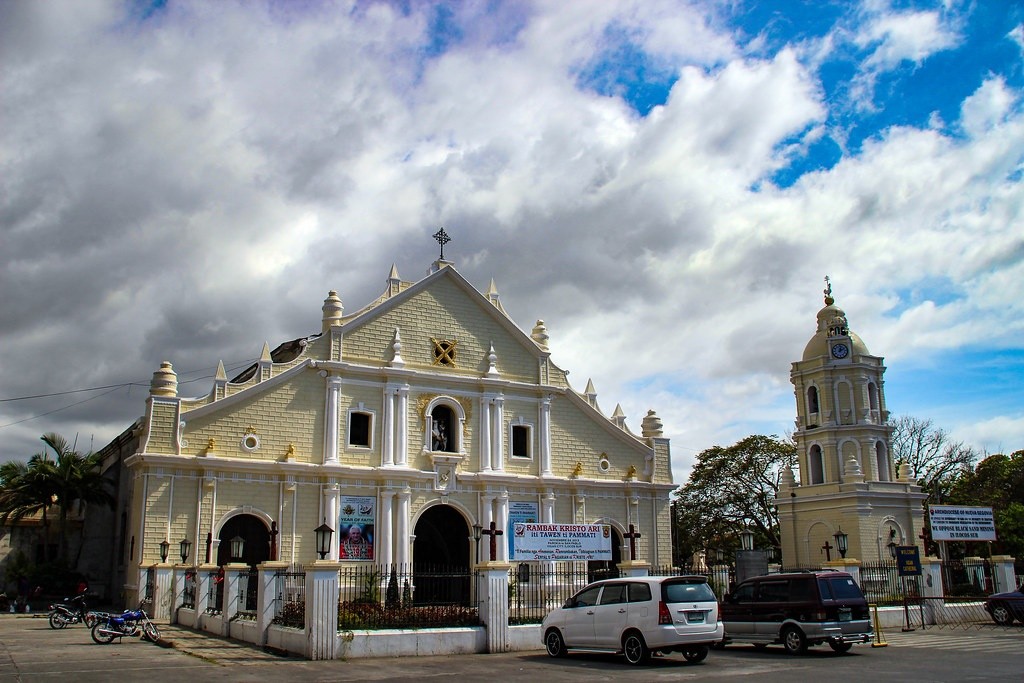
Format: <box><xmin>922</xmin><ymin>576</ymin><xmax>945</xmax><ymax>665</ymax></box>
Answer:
<box><xmin>760</xmin><ymin>593</ymin><xmax>778</xmax><ymax>602</ymax></box>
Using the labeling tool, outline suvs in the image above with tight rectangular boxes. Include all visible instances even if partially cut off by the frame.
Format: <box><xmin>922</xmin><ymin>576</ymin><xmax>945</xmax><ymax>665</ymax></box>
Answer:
<box><xmin>706</xmin><ymin>566</ymin><xmax>876</xmax><ymax>658</ymax></box>
<box><xmin>539</xmin><ymin>575</ymin><xmax>725</xmax><ymax>667</ymax></box>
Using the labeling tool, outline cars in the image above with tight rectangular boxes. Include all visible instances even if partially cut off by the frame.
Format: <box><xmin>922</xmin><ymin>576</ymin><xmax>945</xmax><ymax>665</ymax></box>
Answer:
<box><xmin>984</xmin><ymin>581</ymin><xmax>1024</xmax><ymax>627</ymax></box>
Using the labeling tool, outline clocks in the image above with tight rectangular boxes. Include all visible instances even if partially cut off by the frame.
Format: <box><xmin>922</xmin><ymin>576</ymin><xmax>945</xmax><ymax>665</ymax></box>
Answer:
<box><xmin>832</xmin><ymin>344</ymin><xmax>848</xmax><ymax>359</ymax></box>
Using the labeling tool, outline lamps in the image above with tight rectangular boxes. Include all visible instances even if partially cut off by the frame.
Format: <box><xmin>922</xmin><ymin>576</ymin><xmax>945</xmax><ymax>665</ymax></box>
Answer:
<box><xmin>887</xmin><ymin>542</ymin><xmax>899</xmax><ymax>560</ymax></box>
<box><xmin>179</xmin><ymin>534</ymin><xmax>192</xmax><ymax>563</ymax></box>
<box><xmin>230</xmin><ymin>536</ymin><xmax>245</xmax><ymax>563</ymax></box>
<box><xmin>767</xmin><ymin>545</ymin><xmax>775</xmax><ymax>564</ymax></box>
<box><xmin>313</xmin><ymin>516</ymin><xmax>335</xmax><ymax>560</ymax></box>
<box><xmin>832</xmin><ymin>525</ymin><xmax>849</xmax><ymax>559</ymax></box>
<box><xmin>716</xmin><ymin>546</ymin><xmax>725</xmax><ymax>564</ymax></box>
<box><xmin>159</xmin><ymin>537</ymin><xmax>170</xmax><ymax>563</ymax></box>
<box><xmin>741</xmin><ymin>528</ymin><xmax>754</xmax><ymax>551</ymax></box>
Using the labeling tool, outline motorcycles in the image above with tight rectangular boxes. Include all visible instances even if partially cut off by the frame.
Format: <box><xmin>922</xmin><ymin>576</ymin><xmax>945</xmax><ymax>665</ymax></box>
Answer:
<box><xmin>87</xmin><ymin>595</ymin><xmax>162</xmax><ymax>645</ymax></box>
<box><xmin>47</xmin><ymin>582</ymin><xmax>96</xmax><ymax>630</ymax></box>
